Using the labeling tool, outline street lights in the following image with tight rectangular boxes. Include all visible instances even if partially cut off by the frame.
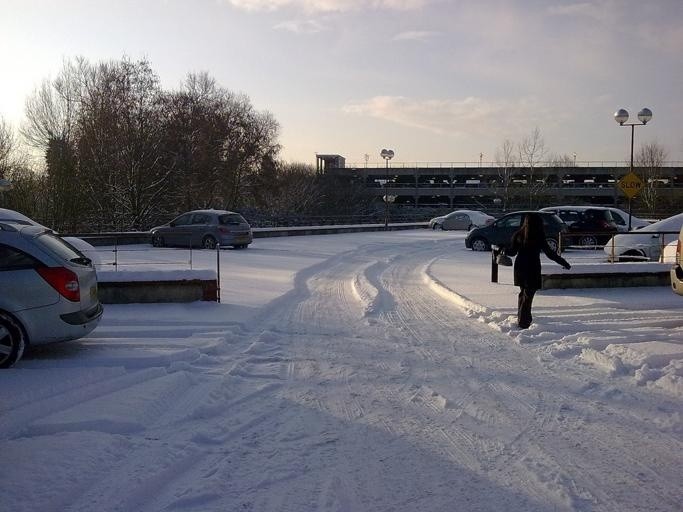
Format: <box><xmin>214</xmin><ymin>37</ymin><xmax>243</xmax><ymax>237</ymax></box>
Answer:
<box><xmin>380</xmin><ymin>149</ymin><xmax>394</xmax><ymax>231</ymax></box>
<box><xmin>614</xmin><ymin>107</ymin><xmax>652</xmax><ymax>231</ymax></box>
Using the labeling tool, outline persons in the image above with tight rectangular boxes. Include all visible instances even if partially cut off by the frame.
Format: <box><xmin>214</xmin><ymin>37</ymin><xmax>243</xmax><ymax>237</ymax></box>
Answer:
<box><xmin>501</xmin><ymin>212</ymin><xmax>571</xmax><ymax>328</ymax></box>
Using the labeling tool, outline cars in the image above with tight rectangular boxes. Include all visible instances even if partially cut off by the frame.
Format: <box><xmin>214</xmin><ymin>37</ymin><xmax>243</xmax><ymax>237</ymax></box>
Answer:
<box><xmin>0</xmin><ymin>218</ymin><xmax>103</xmax><ymax>368</ymax></box>
<box><xmin>538</xmin><ymin>205</ymin><xmax>616</xmax><ymax>245</ymax></box>
<box><xmin>148</xmin><ymin>209</ymin><xmax>252</xmax><ymax>250</ymax></box>
<box><xmin>464</xmin><ymin>211</ymin><xmax>568</xmax><ymax>253</ymax></box>
<box><xmin>608</xmin><ymin>207</ymin><xmax>652</xmax><ymax>233</ymax></box>
<box><xmin>604</xmin><ymin>213</ymin><xmax>682</xmax><ymax>262</ymax></box>
<box><xmin>428</xmin><ymin>210</ymin><xmax>497</xmax><ymax>231</ymax></box>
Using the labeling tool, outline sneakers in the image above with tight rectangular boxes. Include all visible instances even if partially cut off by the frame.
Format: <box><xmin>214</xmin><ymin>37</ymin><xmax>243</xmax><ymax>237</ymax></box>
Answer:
<box><xmin>518</xmin><ymin>315</ymin><xmax>533</xmax><ymax>329</ymax></box>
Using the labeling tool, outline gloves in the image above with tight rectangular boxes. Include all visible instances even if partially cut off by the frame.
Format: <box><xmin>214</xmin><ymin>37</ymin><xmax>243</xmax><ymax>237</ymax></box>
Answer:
<box><xmin>558</xmin><ymin>257</ymin><xmax>572</xmax><ymax>270</ymax></box>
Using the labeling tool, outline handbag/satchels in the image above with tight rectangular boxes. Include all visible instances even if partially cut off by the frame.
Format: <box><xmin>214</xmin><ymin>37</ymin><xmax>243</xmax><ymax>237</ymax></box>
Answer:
<box><xmin>496</xmin><ymin>249</ymin><xmax>512</xmax><ymax>266</ymax></box>
